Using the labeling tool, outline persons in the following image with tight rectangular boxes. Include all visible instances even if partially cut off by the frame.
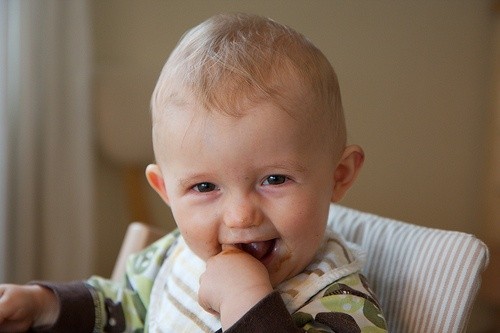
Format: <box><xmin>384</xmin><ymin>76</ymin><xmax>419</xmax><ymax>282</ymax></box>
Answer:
<box><xmin>0</xmin><ymin>9</ymin><xmax>391</xmax><ymax>333</ymax></box>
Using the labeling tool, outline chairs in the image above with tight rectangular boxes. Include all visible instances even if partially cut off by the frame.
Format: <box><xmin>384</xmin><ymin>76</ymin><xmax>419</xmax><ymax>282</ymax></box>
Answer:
<box><xmin>105</xmin><ymin>191</ymin><xmax>489</xmax><ymax>333</ymax></box>
